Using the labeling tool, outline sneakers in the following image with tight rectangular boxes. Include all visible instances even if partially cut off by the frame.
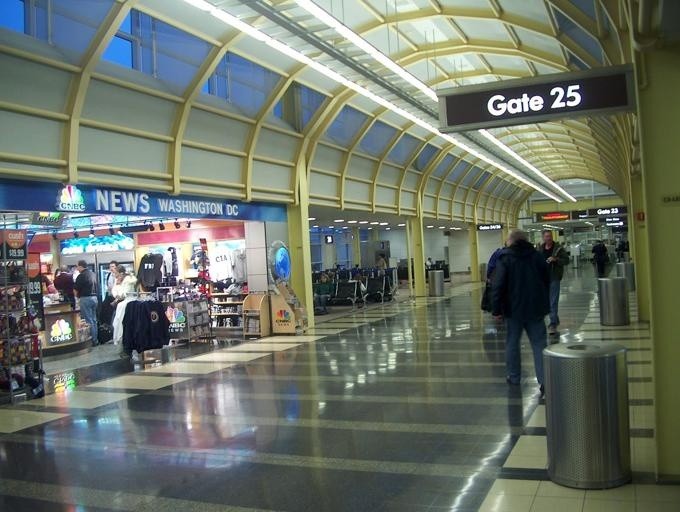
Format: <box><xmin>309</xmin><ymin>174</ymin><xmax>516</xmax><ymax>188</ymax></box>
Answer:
<box><xmin>548</xmin><ymin>326</ymin><xmax>557</xmax><ymax>334</ymax></box>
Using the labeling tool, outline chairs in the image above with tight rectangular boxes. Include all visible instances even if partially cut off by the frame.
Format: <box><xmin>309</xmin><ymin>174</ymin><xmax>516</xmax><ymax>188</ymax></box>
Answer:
<box><xmin>313</xmin><ymin>265</ymin><xmax>398</xmax><ymax>309</ymax></box>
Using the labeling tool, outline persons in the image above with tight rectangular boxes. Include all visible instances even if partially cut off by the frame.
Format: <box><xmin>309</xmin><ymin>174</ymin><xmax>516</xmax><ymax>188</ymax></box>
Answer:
<box><xmin>617</xmin><ymin>240</ymin><xmax>629</xmax><ymax>262</ymax></box>
<box><xmin>480</xmin><ymin>228</ymin><xmax>570</xmax><ymax>394</ymax></box>
<box><xmin>377</xmin><ymin>253</ymin><xmax>388</xmax><ymax>269</ymax></box>
<box><xmin>42</xmin><ymin>260</ymin><xmax>136</xmax><ymax>346</ymax></box>
<box><xmin>347</xmin><ymin>274</ymin><xmax>367</xmax><ymax>294</ymax></box>
<box><xmin>314</xmin><ymin>272</ymin><xmax>335</xmax><ymax>313</ymax></box>
<box><xmin>591</xmin><ymin>239</ymin><xmax>607</xmax><ymax>276</ymax></box>
<box><xmin>508</xmin><ymin>384</ymin><xmax>524</xmax><ymax>438</ymax></box>
<box><xmin>426</xmin><ymin>257</ymin><xmax>432</xmax><ymax>266</ymax></box>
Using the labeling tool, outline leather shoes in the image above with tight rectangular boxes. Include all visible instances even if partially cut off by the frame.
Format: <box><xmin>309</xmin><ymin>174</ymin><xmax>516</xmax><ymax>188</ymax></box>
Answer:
<box><xmin>506</xmin><ymin>373</ymin><xmax>545</xmax><ymax>400</ymax></box>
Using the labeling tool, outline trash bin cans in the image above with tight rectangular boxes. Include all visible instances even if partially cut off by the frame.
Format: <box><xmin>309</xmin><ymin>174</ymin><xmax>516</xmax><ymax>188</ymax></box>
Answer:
<box><xmin>616</xmin><ymin>262</ymin><xmax>634</xmax><ymax>292</ymax></box>
<box><xmin>428</xmin><ymin>270</ymin><xmax>444</xmax><ymax>296</ymax></box>
<box><xmin>598</xmin><ymin>277</ymin><xmax>628</xmax><ymax>326</ymax></box>
<box><xmin>543</xmin><ymin>342</ymin><xmax>631</xmax><ymax>489</ymax></box>
<box><xmin>481</xmin><ymin>263</ymin><xmax>488</xmax><ymax>281</ymax></box>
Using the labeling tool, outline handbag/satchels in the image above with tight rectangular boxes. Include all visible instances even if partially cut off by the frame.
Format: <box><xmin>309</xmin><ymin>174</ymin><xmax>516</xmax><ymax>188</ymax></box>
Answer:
<box><xmin>97</xmin><ymin>321</ymin><xmax>113</xmax><ymax>343</ymax></box>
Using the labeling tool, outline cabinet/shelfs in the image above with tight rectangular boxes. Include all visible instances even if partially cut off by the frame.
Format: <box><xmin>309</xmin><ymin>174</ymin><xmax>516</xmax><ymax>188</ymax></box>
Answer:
<box><xmin>210</xmin><ymin>292</ymin><xmax>271</xmax><ymax>338</ymax></box>
<box><xmin>0</xmin><ymin>213</ymin><xmax>44</xmax><ymax>405</ymax></box>
